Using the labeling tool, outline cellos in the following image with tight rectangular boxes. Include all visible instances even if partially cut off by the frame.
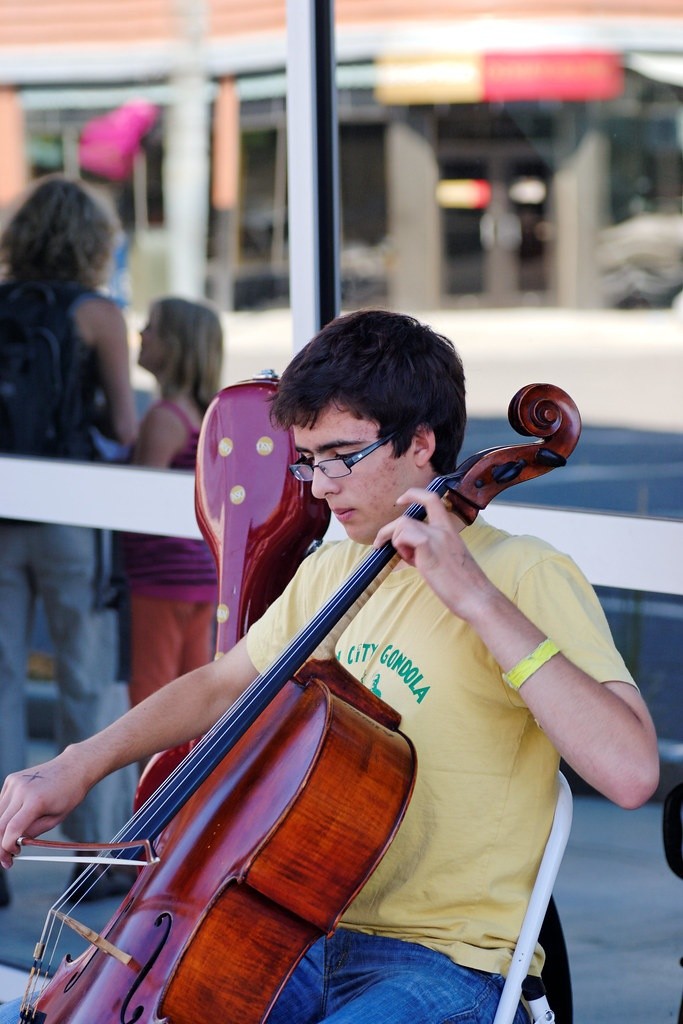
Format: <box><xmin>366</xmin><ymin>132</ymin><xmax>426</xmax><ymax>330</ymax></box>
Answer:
<box><xmin>13</xmin><ymin>385</ymin><xmax>581</xmax><ymax>1023</ymax></box>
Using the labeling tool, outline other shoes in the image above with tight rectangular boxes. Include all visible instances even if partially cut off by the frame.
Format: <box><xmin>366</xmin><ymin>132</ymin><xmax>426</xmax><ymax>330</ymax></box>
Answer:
<box><xmin>65</xmin><ymin>864</ymin><xmax>137</xmax><ymax>904</ymax></box>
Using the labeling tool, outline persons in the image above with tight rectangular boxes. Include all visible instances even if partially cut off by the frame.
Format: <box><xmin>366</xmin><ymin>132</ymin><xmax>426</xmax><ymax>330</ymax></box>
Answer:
<box><xmin>120</xmin><ymin>296</ymin><xmax>229</xmax><ymax>705</ymax></box>
<box><xmin>0</xmin><ymin>176</ymin><xmax>142</xmax><ymax>915</ymax></box>
<box><xmin>0</xmin><ymin>306</ymin><xmax>662</xmax><ymax>1023</ymax></box>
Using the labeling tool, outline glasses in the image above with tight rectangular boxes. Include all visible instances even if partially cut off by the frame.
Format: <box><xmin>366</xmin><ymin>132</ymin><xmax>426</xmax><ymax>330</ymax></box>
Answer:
<box><xmin>289</xmin><ymin>431</ymin><xmax>394</xmax><ymax>482</ymax></box>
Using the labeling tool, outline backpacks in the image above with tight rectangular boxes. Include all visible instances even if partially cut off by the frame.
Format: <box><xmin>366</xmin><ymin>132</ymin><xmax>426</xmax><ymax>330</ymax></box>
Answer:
<box><xmin>0</xmin><ymin>282</ymin><xmax>101</xmax><ymax>460</ymax></box>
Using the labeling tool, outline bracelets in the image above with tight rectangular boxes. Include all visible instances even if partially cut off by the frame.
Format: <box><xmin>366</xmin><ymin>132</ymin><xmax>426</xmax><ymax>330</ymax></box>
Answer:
<box><xmin>501</xmin><ymin>637</ymin><xmax>562</xmax><ymax>693</ymax></box>
